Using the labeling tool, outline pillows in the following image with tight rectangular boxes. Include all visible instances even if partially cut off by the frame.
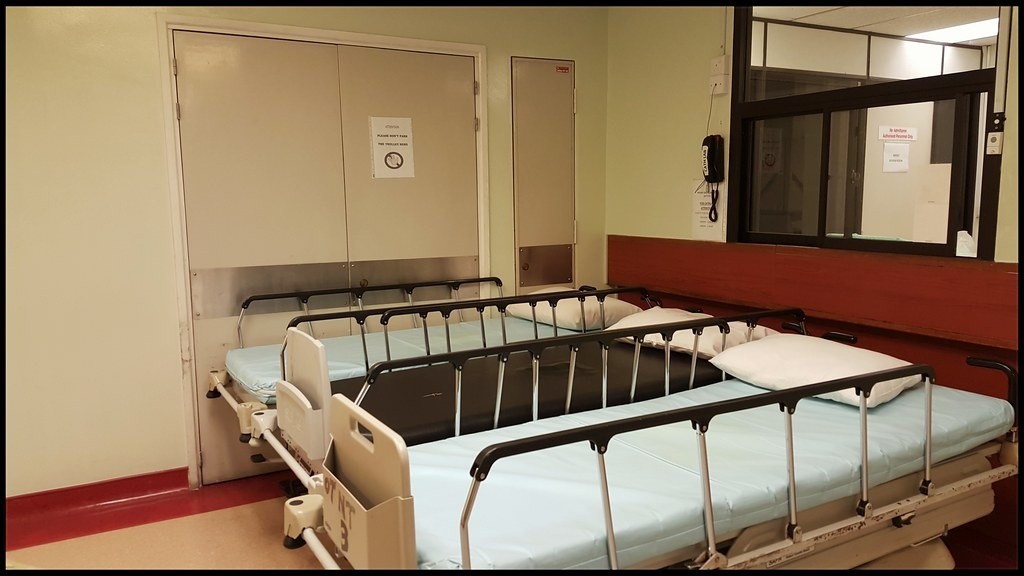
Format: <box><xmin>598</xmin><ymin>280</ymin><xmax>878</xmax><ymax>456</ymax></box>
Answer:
<box><xmin>603</xmin><ymin>304</ymin><xmax>783</xmax><ymax>361</ymax></box>
<box><xmin>505</xmin><ymin>285</ymin><xmax>642</xmax><ymax>333</ymax></box>
<box><xmin>708</xmin><ymin>332</ymin><xmax>922</xmax><ymax>408</ymax></box>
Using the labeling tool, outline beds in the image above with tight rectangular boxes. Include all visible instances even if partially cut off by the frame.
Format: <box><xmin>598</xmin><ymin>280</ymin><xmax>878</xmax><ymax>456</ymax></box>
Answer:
<box><xmin>274</xmin><ymin>276</ymin><xmax>818</xmax><ymax>556</ymax></box>
<box><xmin>285</xmin><ymin>304</ymin><xmax>1018</xmax><ymax>571</ymax></box>
<box><xmin>210</xmin><ymin>275</ymin><xmax>657</xmax><ymax>475</ymax></box>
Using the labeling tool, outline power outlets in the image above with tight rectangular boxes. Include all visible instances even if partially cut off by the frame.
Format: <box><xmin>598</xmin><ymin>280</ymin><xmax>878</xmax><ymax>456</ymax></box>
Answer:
<box><xmin>708</xmin><ymin>56</ymin><xmax>727</xmax><ymax>75</ymax></box>
<box><xmin>707</xmin><ymin>74</ymin><xmax>731</xmax><ymax>95</ymax></box>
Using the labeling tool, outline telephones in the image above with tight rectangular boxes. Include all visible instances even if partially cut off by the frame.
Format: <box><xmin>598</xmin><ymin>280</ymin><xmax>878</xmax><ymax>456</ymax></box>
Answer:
<box><xmin>702</xmin><ymin>134</ymin><xmax>724</xmax><ymax>184</ymax></box>
